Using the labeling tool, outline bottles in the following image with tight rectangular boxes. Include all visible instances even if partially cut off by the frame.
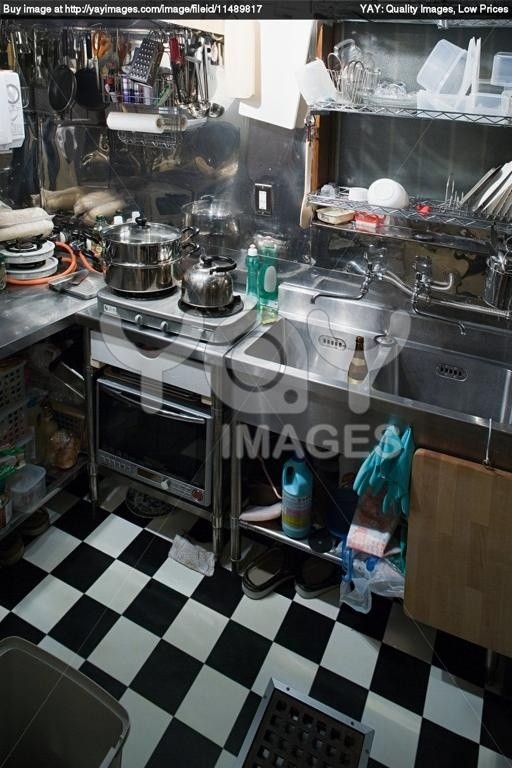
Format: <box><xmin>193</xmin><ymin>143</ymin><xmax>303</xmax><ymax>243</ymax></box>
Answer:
<box><xmin>121</xmin><ymin>73</ymin><xmax>175</xmax><ymax>107</ymax></box>
<box><xmin>38</xmin><ymin>404</ymin><xmax>57</xmax><ymax>463</ymax></box>
<box><xmin>91</xmin><ymin>211</ymin><xmax>142</xmax><ymax>271</ymax></box>
<box><xmin>348</xmin><ymin>336</ymin><xmax>370</xmax><ymax>386</ymax></box>
<box><xmin>246</xmin><ymin>235</ymin><xmax>280</xmax><ymax>325</ymax></box>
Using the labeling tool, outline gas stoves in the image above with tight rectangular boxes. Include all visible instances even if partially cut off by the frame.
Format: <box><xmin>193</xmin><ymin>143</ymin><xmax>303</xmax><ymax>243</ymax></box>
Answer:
<box><xmin>99</xmin><ymin>280</ymin><xmax>261</xmax><ymax>345</ymax></box>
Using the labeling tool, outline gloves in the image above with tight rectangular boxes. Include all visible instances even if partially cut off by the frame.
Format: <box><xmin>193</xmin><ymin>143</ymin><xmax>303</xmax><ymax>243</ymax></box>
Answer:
<box><xmin>352</xmin><ymin>426</ymin><xmax>403</xmax><ymax>499</ymax></box>
<box><xmin>382</xmin><ymin>427</ymin><xmax>415</xmax><ymax>517</ymax></box>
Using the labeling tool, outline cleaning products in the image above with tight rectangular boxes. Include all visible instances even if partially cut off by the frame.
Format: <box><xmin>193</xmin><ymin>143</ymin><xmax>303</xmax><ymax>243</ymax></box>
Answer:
<box><xmin>244</xmin><ymin>244</ymin><xmax>281</xmax><ymax>325</ymax></box>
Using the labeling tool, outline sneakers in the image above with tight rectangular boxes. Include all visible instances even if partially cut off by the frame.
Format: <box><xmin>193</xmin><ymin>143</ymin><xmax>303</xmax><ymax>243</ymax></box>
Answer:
<box><xmin>241</xmin><ymin>543</ymin><xmax>303</xmax><ymax>600</ymax></box>
<box><xmin>294</xmin><ymin>558</ymin><xmax>340</xmax><ymax>599</ymax></box>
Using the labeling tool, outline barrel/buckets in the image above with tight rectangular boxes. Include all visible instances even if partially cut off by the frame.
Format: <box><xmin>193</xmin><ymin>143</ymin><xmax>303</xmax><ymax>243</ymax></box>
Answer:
<box><xmin>279</xmin><ymin>453</ymin><xmax>314</xmax><ymax>540</ymax></box>
<box><xmin>366</xmin><ymin>179</ymin><xmax>409</xmax><ymax>207</ymax></box>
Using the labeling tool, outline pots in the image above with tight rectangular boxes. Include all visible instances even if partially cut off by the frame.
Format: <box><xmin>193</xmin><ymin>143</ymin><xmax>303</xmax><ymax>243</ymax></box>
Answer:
<box><xmin>50</xmin><ymin>30</ymin><xmax>111</xmax><ymax>110</ymax></box>
<box><xmin>99</xmin><ymin>216</ymin><xmax>199</xmax><ymax>292</ymax></box>
<box><xmin>182</xmin><ymin>196</ymin><xmax>241</xmax><ymax>252</ymax></box>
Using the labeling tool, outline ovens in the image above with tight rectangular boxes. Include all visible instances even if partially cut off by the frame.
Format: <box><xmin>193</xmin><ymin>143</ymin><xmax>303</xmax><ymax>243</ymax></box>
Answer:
<box><xmin>91</xmin><ymin>368</ymin><xmax>213</xmax><ymax>509</ymax></box>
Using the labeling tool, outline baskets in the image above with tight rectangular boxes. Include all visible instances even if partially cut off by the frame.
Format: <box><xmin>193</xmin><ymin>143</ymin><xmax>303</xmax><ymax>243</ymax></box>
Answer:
<box><xmin>0</xmin><ymin>359</ymin><xmax>86</xmax><ymax>464</ymax></box>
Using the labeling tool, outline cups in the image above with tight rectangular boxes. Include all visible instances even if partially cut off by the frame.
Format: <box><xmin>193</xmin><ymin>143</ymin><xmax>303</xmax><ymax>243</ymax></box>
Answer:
<box><xmin>348</xmin><ymin>186</ymin><xmax>370</xmax><ymax>203</ymax></box>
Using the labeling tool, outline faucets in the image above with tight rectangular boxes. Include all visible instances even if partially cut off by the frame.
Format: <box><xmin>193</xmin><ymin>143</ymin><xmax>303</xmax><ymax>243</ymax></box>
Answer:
<box><xmin>407</xmin><ymin>254</ymin><xmax>471</xmax><ymax>337</ymax></box>
<box><xmin>309</xmin><ymin>243</ymin><xmax>390</xmax><ymax>306</ymax></box>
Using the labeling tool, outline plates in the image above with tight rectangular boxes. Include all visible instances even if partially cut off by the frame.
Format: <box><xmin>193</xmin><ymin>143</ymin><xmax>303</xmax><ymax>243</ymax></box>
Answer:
<box><xmin>456</xmin><ymin>160</ymin><xmax>512</xmax><ymax>224</ymax></box>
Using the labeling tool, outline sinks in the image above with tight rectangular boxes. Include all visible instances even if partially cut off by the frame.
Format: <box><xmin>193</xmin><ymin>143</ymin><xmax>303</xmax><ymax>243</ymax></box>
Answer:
<box><xmin>370</xmin><ymin>330</ymin><xmax>512</xmax><ymax>429</ymax></box>
<box><xmin>239</xmin><ymin>309</ymin><xmax>385</xmax><ymax>389</ymax></box>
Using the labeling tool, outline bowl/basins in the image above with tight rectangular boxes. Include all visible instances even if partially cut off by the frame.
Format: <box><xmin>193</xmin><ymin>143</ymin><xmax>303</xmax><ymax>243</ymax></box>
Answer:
<box><xmin>124</xmin><ymin>487</ymin><xmax>176</xmax><ymax>517</ymax></box>
<box><xmin>384</xmin><ymin>83</ymin><xmax>407</xmax><ymax>98</ymax></box>
<box><xmin>368</xmin><ymin>178</ymin><xmax>409</xmax><ymax>213</ymax></box>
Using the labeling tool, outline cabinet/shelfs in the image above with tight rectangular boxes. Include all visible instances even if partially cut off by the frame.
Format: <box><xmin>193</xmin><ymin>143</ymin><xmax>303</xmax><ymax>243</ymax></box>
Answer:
<box><xmin>298</xmin><ymin>19</ymin><xmax>512</xmax><ymax>256</ymax></box>
<box><xmin>3</xmin><ymin>323</ymin><xmax>96</xmax><ymax>542</ymax></box>
<box><xmin>227</xmin><ymin>420</ymin><xmax>405</xmax><ymax>593</ymax></box>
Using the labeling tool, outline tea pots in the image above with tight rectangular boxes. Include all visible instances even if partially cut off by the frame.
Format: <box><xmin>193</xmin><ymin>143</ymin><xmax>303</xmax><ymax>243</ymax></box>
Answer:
<box><xmin>180</xmin><ymin>253</ymin><xmax>237</xmax><ymax>309</ymax></box>
